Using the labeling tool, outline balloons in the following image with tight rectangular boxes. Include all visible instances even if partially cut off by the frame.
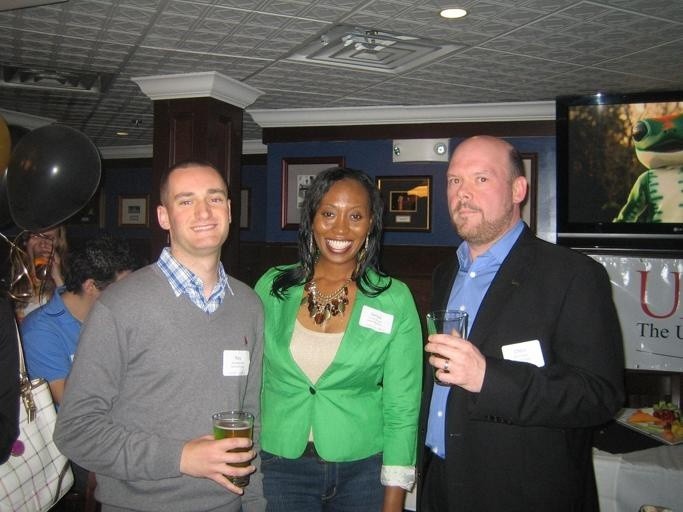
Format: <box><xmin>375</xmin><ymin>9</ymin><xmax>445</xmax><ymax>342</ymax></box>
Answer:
<box><xmin>6</xmin><ymin>125</ymin><xmax>102</xmax><ymax>233</ymax></box>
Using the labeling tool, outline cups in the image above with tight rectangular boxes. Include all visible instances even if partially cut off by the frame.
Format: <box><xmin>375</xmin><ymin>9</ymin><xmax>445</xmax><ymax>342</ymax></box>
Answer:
<box><xmin>424</xmin><ymin>310</ymin><xmax>467</xmax><ymax>384</ymax></box>
<box><xmin>211</xmin><ymin>411</ymin><xmax>254</xmax><ymax>487</ymax></box>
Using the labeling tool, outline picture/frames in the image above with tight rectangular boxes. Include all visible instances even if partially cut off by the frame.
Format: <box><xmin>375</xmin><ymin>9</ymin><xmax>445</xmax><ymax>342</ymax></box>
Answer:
<box><xmin>516</xmin><ymin>152</ymin><xmax>538</xmax><ymax>234</ymax></box>
<box><xmin>373</xmin><ymin>175</ymin><xmax>433</xmax><ymax>235</ymax></box>
<box><xmin>238</xmin><ymin>188</ymin><xmax>250</xmax><ymax>230</ymax></box>
<box><xmin>113</xmin><ymin>192</ymin><xmax>151</xmax><ymax>229</ymax></box>
<box><xmin>278</xmin><ymin>155</ymin><xmax>344</xmax><ymax>232</ymax></box>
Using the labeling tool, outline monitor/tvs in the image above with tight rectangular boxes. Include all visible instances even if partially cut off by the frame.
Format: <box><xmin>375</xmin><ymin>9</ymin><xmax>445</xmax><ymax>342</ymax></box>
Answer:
<box><xmin>556</xmin><ymin>90</ymin><xmax>683</xmax><ymax>235</ymax></box>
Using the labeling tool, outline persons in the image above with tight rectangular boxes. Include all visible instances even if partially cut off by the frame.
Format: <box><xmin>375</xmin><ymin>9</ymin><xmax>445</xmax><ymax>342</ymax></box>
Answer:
<box><xmin>0</xmin><ymin>294</ymin><xmax>20</xmax><ymax>466</ymax></box>
<box><xmin>19</xmin><ymin>228</ymin><xmax>148</xmax><ymax>488</ymax></box>
<box><xmin>8</xmin><ymin>222</ymin><xmax>75</xmax><ymax>324</ymax></box>
<box><xmin>416</xmin><ymin>132</ymin><xmax>628</xmax><ymax>511</ymax></box>
<box><xmin>251</xmin><ymin>164</ymin><xmax>427</xmax><ymax>511</ymax></box>
<box><xmin>51</xmin><ymin>156</ymin><xmax>269</xmax><ymax>512</ymax></box>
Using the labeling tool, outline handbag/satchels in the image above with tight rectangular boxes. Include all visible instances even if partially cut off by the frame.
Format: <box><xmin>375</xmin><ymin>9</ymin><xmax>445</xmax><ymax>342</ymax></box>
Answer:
<box><xmin>0</xmin><ymin>311</ymin><xmax>76</xmax><ymax>512</ymax></box>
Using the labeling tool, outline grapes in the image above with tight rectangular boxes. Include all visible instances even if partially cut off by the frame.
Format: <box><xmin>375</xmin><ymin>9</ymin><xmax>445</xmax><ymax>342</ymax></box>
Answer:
<box><xmin>653</xmin><ymin>409</ymin><xmax>674</xmax><ymax>421</ymax></box>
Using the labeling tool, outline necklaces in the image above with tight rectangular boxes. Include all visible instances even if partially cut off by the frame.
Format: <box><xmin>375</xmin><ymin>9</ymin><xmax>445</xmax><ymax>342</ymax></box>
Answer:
<box><xmin>298</xmin><ymin>257</ymin><xmax>353</xmax><ymax>328</ymax></box>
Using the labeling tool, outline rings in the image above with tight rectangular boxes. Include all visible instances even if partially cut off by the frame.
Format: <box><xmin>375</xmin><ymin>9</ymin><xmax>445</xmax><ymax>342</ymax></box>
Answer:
<box><xmin>444</xmin><ymin>358</ymin><xmax>451</xmax><ymax>371</ymax></box>
